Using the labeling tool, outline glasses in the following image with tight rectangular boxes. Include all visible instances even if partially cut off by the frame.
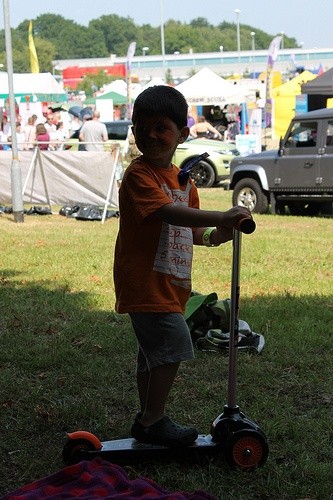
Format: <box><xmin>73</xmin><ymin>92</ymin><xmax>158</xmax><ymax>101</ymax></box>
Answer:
<box><xmin>47</xmin><ymin>118</ymin><xmax>53</xmax><ymax>120</ymax></box>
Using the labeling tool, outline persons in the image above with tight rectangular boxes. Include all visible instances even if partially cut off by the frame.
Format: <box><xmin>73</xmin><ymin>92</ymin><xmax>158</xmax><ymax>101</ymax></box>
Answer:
<box><xmin>113</xmin><ymin>84</ymin><xmax>252</xmax><ymax>443</ymax></box>
<box><xmin>209</xmin><ymin>106</ymin><xmax>229</xmax><ymax>135</ymax></box>
<box><xmin>0</xmin><ymin>107</ymin><xmax>108</xmax><ymax>151</ymax></box>
<box><xmin>189</xmin><ymin>116</ymin><xmax>223</xmax><ymax>138</ymax></box>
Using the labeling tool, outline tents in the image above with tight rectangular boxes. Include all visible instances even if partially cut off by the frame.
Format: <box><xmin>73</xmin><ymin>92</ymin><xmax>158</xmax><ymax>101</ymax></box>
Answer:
<box><xmin>94</xmin><ymin>68</ymin><xmax>262</xmax><ymax>145</ymax></box>
<box><xmin>271</xmin><ymin>69</ymin><xmax>333</xmax><ymax>140</ymax></box>
<box><xmin>0</xmin><ymin>72</ymin><xmax>67</xmax><ymax>106</ymax></box>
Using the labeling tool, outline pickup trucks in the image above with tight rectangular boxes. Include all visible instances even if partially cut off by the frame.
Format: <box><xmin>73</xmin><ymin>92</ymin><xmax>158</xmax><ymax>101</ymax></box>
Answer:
<box><xmin>62</xmin><ymin>124</ymin><xmax>241</xmax><ymax>188</ymax></box>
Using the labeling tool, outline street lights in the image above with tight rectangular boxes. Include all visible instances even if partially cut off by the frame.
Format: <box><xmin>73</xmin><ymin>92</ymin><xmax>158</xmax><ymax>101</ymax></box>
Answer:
<box><xmin>233</xmin><ymin>9</ymin><xmax>244</xmax><ymax>72</ymax></box>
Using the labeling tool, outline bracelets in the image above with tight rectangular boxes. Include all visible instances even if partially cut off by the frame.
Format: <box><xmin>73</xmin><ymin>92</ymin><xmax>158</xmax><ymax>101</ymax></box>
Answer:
<box><xmin>202</xmin><ymin>228</ymin><xmax>221</xmax><ymax>247</ymax></box>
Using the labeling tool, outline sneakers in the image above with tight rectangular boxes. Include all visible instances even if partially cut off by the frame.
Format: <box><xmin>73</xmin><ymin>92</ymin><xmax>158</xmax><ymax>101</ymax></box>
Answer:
<box><xmin>130</xmin><ymin>411</ymin><xmax>198</xmax><ymax>444</ymax></box>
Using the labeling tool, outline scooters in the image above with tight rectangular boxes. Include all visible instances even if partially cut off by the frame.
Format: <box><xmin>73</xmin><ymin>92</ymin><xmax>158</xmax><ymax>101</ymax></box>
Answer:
<box><xmin>62</xmin><ymin>219</ymin><xmax>270</xmax><ymax>469</ymax></box>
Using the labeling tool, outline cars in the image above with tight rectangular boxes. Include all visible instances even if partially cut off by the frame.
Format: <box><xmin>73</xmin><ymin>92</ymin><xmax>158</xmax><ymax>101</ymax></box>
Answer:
<box><xmin>224</xmin><ymin>108</ymin><xmax>333</xmax><ymax>215</ymax></box>
<box><xmin>65</xmin><ymin>120</ymin><xmax>132</xmax><ymax>148</ymax></box>
